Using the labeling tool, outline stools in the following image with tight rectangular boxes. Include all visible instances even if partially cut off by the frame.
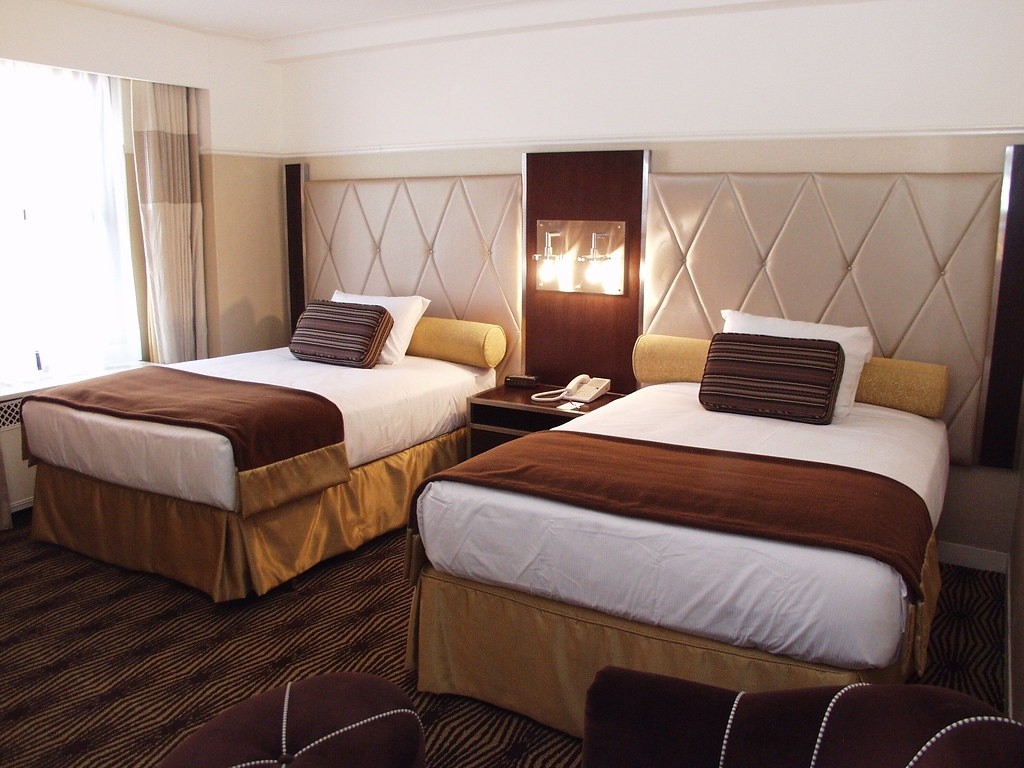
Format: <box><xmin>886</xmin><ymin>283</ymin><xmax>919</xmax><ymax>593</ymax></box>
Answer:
<box><xmin>157</xmin><ymin>672</ymin><xmax>428</xmax><ymax>767</ymax></box>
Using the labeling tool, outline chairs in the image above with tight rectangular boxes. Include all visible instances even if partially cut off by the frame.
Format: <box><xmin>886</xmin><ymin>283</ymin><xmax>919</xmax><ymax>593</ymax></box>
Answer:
<box><xmin>586</xmin><ymin>653</ymin><xmax>1024</xmax><ymax>766</ymax></box>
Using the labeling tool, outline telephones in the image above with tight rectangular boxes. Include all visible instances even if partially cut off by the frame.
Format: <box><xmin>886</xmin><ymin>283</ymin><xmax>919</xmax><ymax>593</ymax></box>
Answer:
<box><xmin>562</xmin><ymin>373</ymin><xmax>611</xmax><ymax>404</ymax></box>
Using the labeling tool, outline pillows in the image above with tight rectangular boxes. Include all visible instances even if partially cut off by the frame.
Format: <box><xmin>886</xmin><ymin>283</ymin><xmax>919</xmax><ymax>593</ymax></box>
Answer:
<box><xmin>698</xmin><ymin>333</ymin><xmax>845</xmax><ymax>424</ymax></box>
<box><xmin>720</xmin><ymin>309</ymin><xmax>874</xmax><ymax>419</ymax></box>
<box><xmin>404</xmin><ymin>317</ymin><xmax>507</xmax><ymax>368</ymax></box>
<box><xmin>289</xmin><ymin>297</ymin><xmax>394</xmax><ymax>368</ymax></box>
<box><xmin>632</xmin><ymin>333</ymin><xmax>949</xmax><ymax>420</ymax></box>
<box><xmin>331</xmin><ymin>290</ymin><xmax>432</xmax><ymax>365</ymax></box>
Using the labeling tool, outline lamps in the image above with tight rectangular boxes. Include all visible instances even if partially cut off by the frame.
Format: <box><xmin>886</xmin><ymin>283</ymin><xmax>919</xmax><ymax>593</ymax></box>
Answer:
<box><xmin>531</xmin><ymin>230</ymin><xmax>610</xmax><ymax>284</ymax></box>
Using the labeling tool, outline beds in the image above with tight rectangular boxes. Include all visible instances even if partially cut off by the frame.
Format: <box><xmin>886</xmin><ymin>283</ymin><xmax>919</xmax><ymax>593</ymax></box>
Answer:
<box><xmin>19</xmin><ymin>346</ymin><xmax>498</xmax><ymax>604</ymax></box>
<box><xmin>400</xmin><ymin>378</ymin><xmax>952</xmax><ymax>745</ymax></box>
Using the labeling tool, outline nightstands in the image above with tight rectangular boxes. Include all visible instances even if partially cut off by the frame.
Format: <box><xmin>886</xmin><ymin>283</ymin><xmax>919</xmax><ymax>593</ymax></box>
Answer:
<box><xmin>468</xmin><ymin>380</ymin><xmax>628</xmax><ymax>461</ymax></box>
